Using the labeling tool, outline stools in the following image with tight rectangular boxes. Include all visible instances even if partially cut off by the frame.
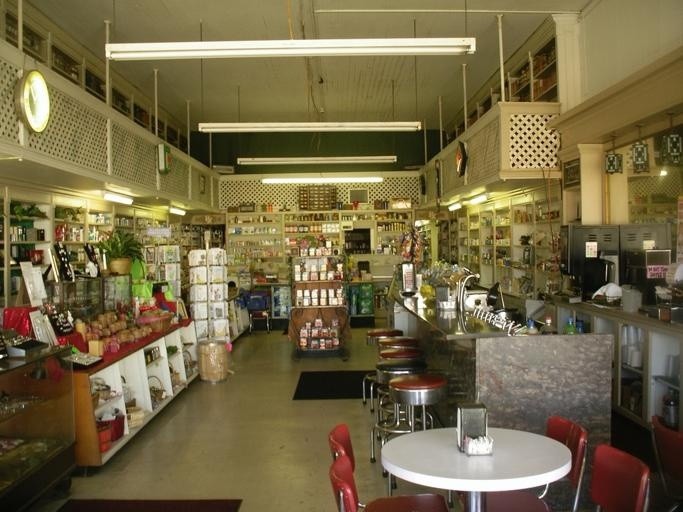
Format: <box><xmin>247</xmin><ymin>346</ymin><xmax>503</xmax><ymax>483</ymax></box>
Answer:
<box><xmin>377</xmin><ymin>348</ymin><xmax>423</xmax><ymax>441</ymax></box>
<box><xmin>377</xmin><ymin>338</ymin><xmax>419</xmax><ymax>406</ymax></box>
<box><xmin>364</xmin><ymin>330</ymin><xmax>403</xmax><ymax>413</ymax></box>
<box><xmin>389</xmin><ymin>375</ymin><xmax>453</xmax><ymax>509</ymax></box>
<box><xmin>370</xmin><ymin>359</ymin><xmax>433</xmax><ymax>477</ymax></box>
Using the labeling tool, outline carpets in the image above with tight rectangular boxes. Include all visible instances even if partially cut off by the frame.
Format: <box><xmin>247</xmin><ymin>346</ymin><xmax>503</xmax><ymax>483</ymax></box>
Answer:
<box><xmin>292</xmin><ymin>370</ymin><xmax>388</xmax><ymax>400</ymax></box>
<box><xmin>55</xmin><ymin>499</ymin><xmax>243</xmax><ymax>512</ymax></box>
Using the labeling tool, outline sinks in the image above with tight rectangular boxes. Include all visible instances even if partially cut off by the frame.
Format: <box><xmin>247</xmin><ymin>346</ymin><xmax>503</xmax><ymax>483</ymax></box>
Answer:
<box><xmin>495</xmin><ymin>309</ymin><xmax>546</xmax><ymax>331</ymax></box>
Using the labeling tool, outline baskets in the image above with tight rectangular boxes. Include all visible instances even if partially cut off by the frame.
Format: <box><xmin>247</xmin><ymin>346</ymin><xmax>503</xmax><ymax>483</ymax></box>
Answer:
<box><xmin>185</xmin><ymin>350</ymin><xmax>196</xmax><ymax>368</ymax></box>
<box><xmin>169</xmin><ymin>362</ymin><xmax>180</xmax><ymax>386</ymax></box>
<box><xmin>92</xmin><ymin>391</ymin><xmax>99</xmax><ymax>407</ymax></box>
<box><xmin>149</xmin><ymin>376</ymin><xmax>167</xmax><ymax>401</ymax></box>
<box><xmin>95</xmin><ymin>379</ymin><xmax>111</xmax><ymax>400</ymax></box>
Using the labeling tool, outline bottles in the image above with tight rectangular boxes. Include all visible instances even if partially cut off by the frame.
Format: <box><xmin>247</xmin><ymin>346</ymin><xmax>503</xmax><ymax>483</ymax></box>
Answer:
<box><xmin>342</xmin><ymin>214</ymin><xmax>371</xmax><ymax>222</ymax></box>
<box><xmin>578</xmin><ymin>320</ymin><xmax>584</xmax><ymax>333</ymax></box>
<box><xmin>663</xmin><ymin>390</ymin><xmax>678</xmax><ymax>429</ymax></box>
<box><xmin>538</xmin><ymin>315</ymin><xmax>558</xmax><ymax>335</ymax></box>
<box><xmin>285</xmin><ymin>214</ymin><xmax>339</xmax><ymax>233</ymax></box>
<box><xmin>524</xmin><ymin>320</ymin><xmax>539</xmax><ymax>336</ymax></box>
<box><xmin>563</xmin><ymin>317</ymin><xmax>578</xmax><ymax>336</ymax></box>
<box><xmin>374</xmin><ymin>212</ymin><xmax>408</xmax><ymax>231</ymax></box>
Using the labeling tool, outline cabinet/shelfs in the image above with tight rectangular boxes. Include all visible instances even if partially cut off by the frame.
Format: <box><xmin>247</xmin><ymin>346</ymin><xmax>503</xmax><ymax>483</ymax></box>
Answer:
<box><xmin>226</xmin><ymin>209</ymin><xmax>415</xmax><ymax>258</ymax></box>
<box><xmin>253</xmin><ymin>282</ymin><xmax>393</xmax><ymax>328</ymax></box>
<box><xmin>228</xmin><ymin>294</ymin><xmax>250</xmax><ymax>343</ymax></box>
<box><xmin>0</xmin><ymin>176</ymin><xmax>223</xmax><ymax>307</ymax></box>
<box><xmin>437</xmin><ymin>183</ymin><xmax>563</xmax><ymax>301</ymax></box>
<box><xmin>288</xmin><ymin>257</ymin><xmax>352</xmax><ymax>362</ymax></box>
<box><xmin>63</xmin><ymin>319</ymin><xmax>199</xmax><ymax>478</ymax></box>
<box><xmin>393</xmin><ymin>289</ymin><xmax>613</xmax><ymax>470</ymax></box>
<box><xmin>555</xmin><ymin>297</ymin><xmax>683</xmax><ymax>448</ymax></box>
<box><xmin>0</xmin><ymin>340</ymin><xmax>78</xmax><ymax>512</ymax></box>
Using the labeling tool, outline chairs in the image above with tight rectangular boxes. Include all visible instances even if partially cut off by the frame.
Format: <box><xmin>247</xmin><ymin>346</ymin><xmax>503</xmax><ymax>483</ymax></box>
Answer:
<box><xmin>651</xmin><ymin>416</ymin><xmax>683</xmax><ymax>512</ymax></box>
<box><xmin>248</xmin><ymin>295</ymin><xmax>272</xmax><ymax>334</ymax></box>
<box><xmin>330</xmin><ymin>456</ymin><xmax>358</xmax><ymax>512</ymax></box>
<box><xmin>358</xmin><ymin>261</ymin><xmax>370</xmax><ymax>277</ymax></box>
<box><xmin>329</xmin><ymin>424</ymin><xmax>450</xmax><ymax>512</ymax></box>
<box><xmin>457</xmin><ymin>416</ymin><xmax>589</xmax><ymax>511</ymax></box>
<box><xmin>591</xmin><ymin>444</ymin><xmax>650</xmax><ymax>512</ymax></box>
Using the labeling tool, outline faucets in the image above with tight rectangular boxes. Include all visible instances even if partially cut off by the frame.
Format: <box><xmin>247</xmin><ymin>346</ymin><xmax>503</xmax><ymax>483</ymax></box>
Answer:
<box><xmin>460</xmin><ymin>274</ymin><xmax>481</xmax><ymax>312</ymax></box>
<box><xmin>458</xmin><ymin>276</ymin><xmax>466</xmax><ymax>308</ymax></box>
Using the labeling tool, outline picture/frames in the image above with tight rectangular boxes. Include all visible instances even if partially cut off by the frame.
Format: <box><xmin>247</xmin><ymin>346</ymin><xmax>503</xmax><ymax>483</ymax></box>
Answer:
<box><xmin>457</xmin><ymin>141</ymin><xmax>467</xmax><ymax>176</ymax></box>
<box><xmin>47</xmin><ymin>248</ymin><xmax>61</xmax><ymax>282</ymax></box>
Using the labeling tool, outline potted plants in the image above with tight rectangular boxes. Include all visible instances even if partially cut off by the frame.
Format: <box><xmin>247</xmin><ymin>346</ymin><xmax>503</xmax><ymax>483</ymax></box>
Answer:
<box><xmin>95</xmin><ymin>227</ymin><xmax>151</xmax><ymax>276</ymax></box>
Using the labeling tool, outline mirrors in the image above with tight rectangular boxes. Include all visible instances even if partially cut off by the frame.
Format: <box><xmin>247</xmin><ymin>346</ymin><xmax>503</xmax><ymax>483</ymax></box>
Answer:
<box><xmin>608</xmin><ymin>124</ymin><xmax>683</xmax><ymax>264</ymax></box>
<box><xmin>415</xmin><ymin>220</ymin><xmax>431</xmax><ymax>274</ymax></box>
<box><xmin>349</xmin><ymin>188</ymin><xmax>369</xmax><ymax>205</ymax></box>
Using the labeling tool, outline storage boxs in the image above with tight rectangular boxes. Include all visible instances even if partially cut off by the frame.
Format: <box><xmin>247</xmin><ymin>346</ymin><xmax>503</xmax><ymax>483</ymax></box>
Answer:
<box><xmin>144</xmin><ymin>346</ymin><xmax>160</xmax><ymax>365</ymax></box>
<box><xmin>97</xmin><ymin>422</ymin><xmax>112</xmax><ymax>453</ymax></box>
<box><xmin>102</xmin><ymin>415</ymin><xmax>125</xmax><ymax>441</ymax></box>
<box><xmin>390</xmin><ymin>197</ymin><xmax>411</xmax><ymax>209</ymax></box>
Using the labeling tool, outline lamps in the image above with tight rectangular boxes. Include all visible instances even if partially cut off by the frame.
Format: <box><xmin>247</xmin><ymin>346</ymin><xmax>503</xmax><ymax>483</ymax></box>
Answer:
<box><xmin>14</xmin><ymin>69</ymin><xmax>50</xmax><ymax>133</ymax></box>
<box><xmin>198</xmin><ymin>20</ymin><xmax>422</xmax><ymax>133</ymax></box>
<box><xmin>237</xmin><ymin>81</ymin><xmax>397</xmax><ymax>165</ymax></box>
<box><xmin>105</xmin><ymin>1</ymin><xmax>476</xmax><ymax>60</ymax></box>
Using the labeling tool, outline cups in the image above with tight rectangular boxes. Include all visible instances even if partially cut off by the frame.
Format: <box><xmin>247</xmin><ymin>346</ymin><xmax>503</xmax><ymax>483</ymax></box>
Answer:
<box><xmin>665</xmin><ymin>353</ymin><xmax>682</xmax><ymax>383</ymax></box>
<box><xmin>620</xmin><ymin>324</ymin><xmax>644</xmax><ymax>367</ymax></box>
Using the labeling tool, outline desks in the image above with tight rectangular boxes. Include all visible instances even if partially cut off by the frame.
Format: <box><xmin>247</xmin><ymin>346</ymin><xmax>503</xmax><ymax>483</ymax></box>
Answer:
<box><xmin>381</xmin><ymin>426</ymin><xmax>572</xmax><ymax>512</ymax></box>
